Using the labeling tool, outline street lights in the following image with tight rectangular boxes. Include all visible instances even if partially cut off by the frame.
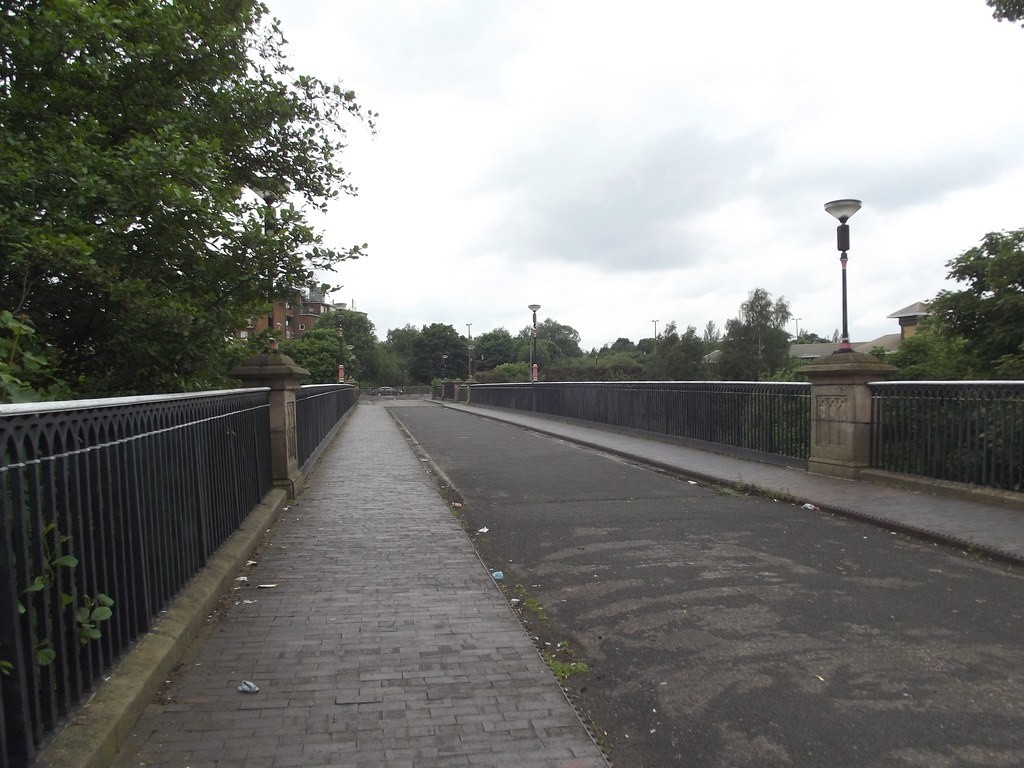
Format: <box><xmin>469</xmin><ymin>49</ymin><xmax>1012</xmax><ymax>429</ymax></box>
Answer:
<box><xmin>399</xmin><ymin>368</ymin><xmax>407</xmax><ymax>395</ymax></box>
<box><xmin>443</xmin><ymin>355</ymin><xmax>448</xmax><ymax>380</ymax></box>
<box><xmin>466</xmin><ymin>323</ymin><xmax>473</xmax><ymax>380</ymax></box>
<box><xmin>652</xmin><ymin>320</ymin><xmax>659</xmax><ymax>341</ymax></box>
<box><xmin>338</xmin><ymin>309</ymin><xmax>346</xmax><ymax>385</ymax></box>
<box><xmin>248</xmin><ymin>174</ymin><xmax>291</xmax><ymax>359</ymax></box>
<box><xmin>528</xmin><ymin>304</ymin><xmax>541</xmax><ymax>373</ymax></box>
<box><xmin>346</xmin><ymin>344</ymin><xmax>356</xmax><ymax>382</ymax></box>
<box><xmin>823</xmin><ymin>198</ymin><xmax>862</xmax><ymax>354</ymax></box>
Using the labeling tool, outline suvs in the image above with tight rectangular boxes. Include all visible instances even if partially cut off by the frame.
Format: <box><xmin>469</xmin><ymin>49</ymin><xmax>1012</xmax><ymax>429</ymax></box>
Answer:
<box><xmin>378</xmin><ymin>387</ymin><xmax>403</xmax><ymax>397</ymax></box>
